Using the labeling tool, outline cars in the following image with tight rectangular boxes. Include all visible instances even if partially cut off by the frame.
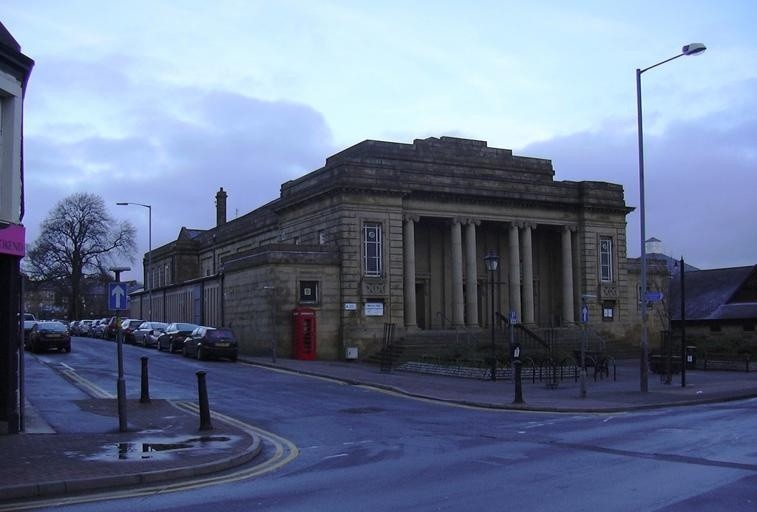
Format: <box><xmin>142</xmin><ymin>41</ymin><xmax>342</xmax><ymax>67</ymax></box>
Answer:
<box><xmin>16</xmin><ymin>311</ymin><xmax>237</xmax><ymax>362</ymax></box>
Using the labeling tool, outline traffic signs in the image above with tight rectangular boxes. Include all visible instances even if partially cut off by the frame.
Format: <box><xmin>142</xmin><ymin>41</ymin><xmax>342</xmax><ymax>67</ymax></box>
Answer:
<box><xmin>643</xmin><ymin>291</ymin><xmax>663</xmax><ymax>301</ymax></box>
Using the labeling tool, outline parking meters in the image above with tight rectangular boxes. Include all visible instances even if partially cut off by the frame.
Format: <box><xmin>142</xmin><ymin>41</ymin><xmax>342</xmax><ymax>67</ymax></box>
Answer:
<box><xmin>510</xmin><ymin>342</ymin><xmax>524</xmax><ymax>403</ymax></box>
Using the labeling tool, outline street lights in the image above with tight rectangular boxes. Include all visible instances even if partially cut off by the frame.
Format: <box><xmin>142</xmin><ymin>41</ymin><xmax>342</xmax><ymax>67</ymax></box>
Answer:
<box><xmin>481</xmin><ymin>248</ymin><xmax>501</xmax><ymax>380</ymax></box>
<box><xmin>633</xmin><ymin>39</ymin><xmax>707</xmax><ymax>390</ymax></box>
<box><xmin>671</xmin><ymin>255</ymin><xmax>685</xmax><ymax>388</ymax></box>
<box><xmin>579</xmin><ymin>294</ymin><xmax>600</xmax><ymax>398</ymax></box>
<box><xmin>116</xmin><ymin>201</ymin><xmax>153</xmax><ymax>321</ymax></box>
<box><xmin>260</xmin><ymin>286</ymin><xmax>277</xmax><ymax>363</ymax></box>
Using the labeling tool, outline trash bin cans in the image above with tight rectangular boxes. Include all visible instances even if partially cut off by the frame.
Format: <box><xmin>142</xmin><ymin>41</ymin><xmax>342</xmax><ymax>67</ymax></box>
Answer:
<box><xmin>685</xmin><ymin>346</ymin><xmax>696</xmax><ymax>368</ymax></box>
